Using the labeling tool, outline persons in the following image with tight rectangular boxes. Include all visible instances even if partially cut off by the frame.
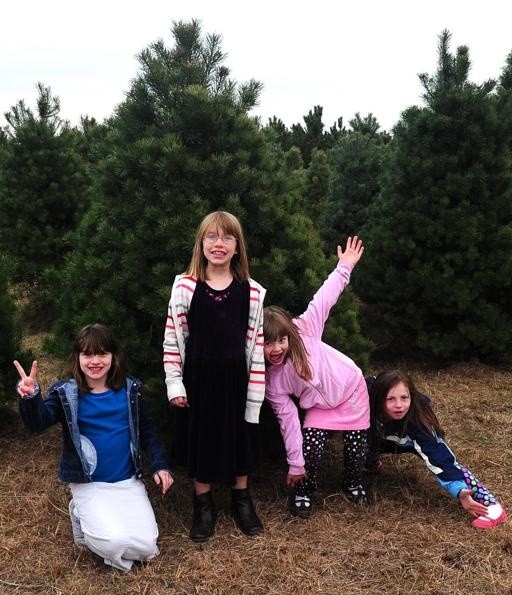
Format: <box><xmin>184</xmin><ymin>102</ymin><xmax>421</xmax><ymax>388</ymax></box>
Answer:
<box><xmin>363</xmin><ymin>369</ymin><xmax>504</xmax><ymax>527</ymax></box>
<box><xmin>13</xmin><ymin>324</ymin><xmax>175</xmax><ymax>574</ymax></box>
<box><xmin>163</xmin><ymin>209</ymin><xmax>268</xmax><ymax>542</ymax></box>
<box><xmin>262</xmin><ymin>235</ymin><xmax>368</xmax><ymax>515</ymax></box>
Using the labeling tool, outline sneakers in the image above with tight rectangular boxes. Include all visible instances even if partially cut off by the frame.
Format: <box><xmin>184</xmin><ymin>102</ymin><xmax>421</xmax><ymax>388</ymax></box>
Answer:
<box><xmin>362</xmin><ymin>461</ymin><xmax>384</xmax><ymax>473</ymax></box>
<box><xmin>69</xmin><ymin>498</ymin><xmax>84</xmax><ymax>538</ymax></box>
<box><xmin>346</xmin><ymin>485</ymin><xmax>371</xmax><ymax>505</ymax></box>
<box><xmin>471</xmin><ymin>507</ymin><xmax>507</xmax><ymax>528</ymax></box>
<box><xmin>290</xmin><ymin>494</ymin><xmax>311</xmax><ymax>517</ymax></box>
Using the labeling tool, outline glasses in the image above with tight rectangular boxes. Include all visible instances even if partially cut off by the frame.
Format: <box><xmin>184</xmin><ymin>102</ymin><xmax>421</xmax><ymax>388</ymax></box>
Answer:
<box><xmin>202</xmin><ymin>234</ymin><xmax>238</xmax><ymax>244</ymax></box>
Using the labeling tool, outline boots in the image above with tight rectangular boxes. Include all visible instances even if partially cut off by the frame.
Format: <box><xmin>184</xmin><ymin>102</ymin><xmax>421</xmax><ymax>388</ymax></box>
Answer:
<box><xmin>231</xmin><ymin>487</ymin><xmax>264</xmax><ymax>535</ymax></box>
<box><xmin>191</xmin><ymin>488</ymin><xmax>215</xmax><ymax>542</ymax></box>
